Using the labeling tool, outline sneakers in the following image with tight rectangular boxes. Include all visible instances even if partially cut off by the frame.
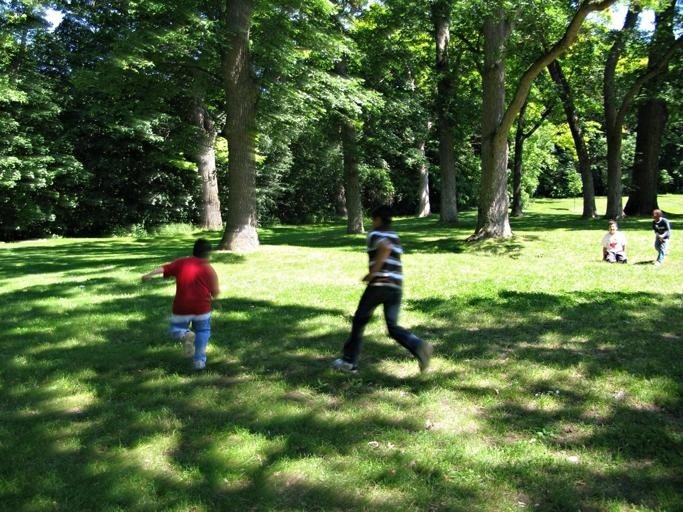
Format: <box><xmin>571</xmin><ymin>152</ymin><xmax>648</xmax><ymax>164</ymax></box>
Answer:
<box><xmin>184</xmin><ymin>331</ymin><xmax>196</xmax><ymax>358</ymax></box>
<box><xmin>417</xmin><ymin>344</ymin><xmax>432</xmax><ymax>373</ymax></box>
<box><xmin>336</xmin><ymin>359</ymin><xmax>358</xmax><ymax>374</ymax></box>
<box><xmin>196</xmin><ymin>360</ymin><xmax>206</xmax><ymax>369</ymax></box>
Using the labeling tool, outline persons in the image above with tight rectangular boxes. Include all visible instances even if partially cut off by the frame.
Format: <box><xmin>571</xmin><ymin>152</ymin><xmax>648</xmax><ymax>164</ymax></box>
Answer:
<box><xmin>602</xmin><ymin>219</ymin><xmax>628</xmax><ymax>264</ymax></box>
<box><xmin>333</xmin><ymin>206</ymin><xmax>434</xmax><ymax>374</ymax></box>
<box><xmin>142</xmin><ymin>238</ymin><xmax>219</xmax><ymax>370</ymax></box>
<box><xmin>652</xmin><ymin>209</ymin><xmax>670</xmax><ymax>266</ymax></box>
